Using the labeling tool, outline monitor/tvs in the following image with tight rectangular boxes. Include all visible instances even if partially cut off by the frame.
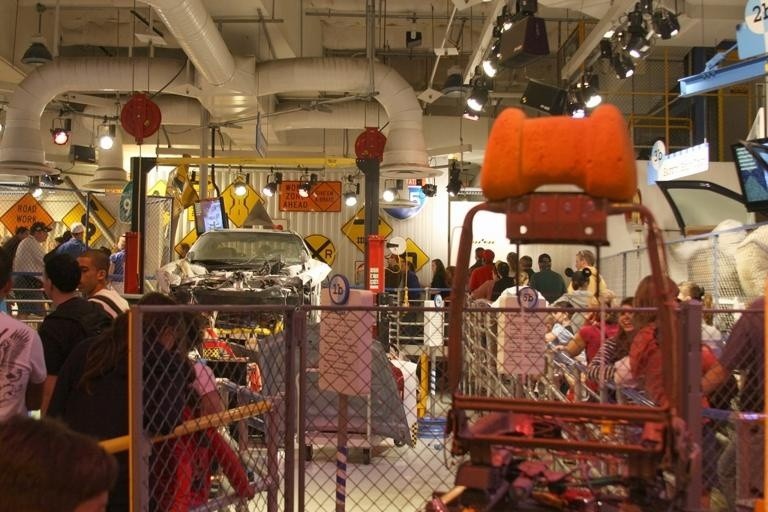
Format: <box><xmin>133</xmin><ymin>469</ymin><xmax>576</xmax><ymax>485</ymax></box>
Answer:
<box><xmin>730</xmin><ymin>138</ymin><xmax>768</xmax><ymax>212</ymax></box>
<box><xmin>192</xmin><ymin>196</ymin><xmax>229</xmax><ymax>237</ymax></box>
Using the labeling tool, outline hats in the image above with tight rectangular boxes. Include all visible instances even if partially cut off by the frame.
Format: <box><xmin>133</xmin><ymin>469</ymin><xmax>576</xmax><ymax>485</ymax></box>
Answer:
<box><xmin>479</xmin><ymin>249</ymin><xmax>495</xmax><ymax>260</ymax></box>
<box><xmin>70</xmin><ymin>223</ymin><xmax>84</xmax><ymax>234</ymax></box>
<box><xmin>32</xmin><ymin>222</ymin><xmax>52</xmax><ymax>232</ymax></box>
<box><xmin>538</xmin><ymin>253</ymin><xmax>551</xmax><ymax>263</ymax></box>
<box><xmin>689</xmin><ymin>286</ymin><xmax>705</xmax><ymax>298</ymax></box>
<box><xmin>385</xmin><ymin>253</ymin><xmax>392</xmax><ymax>259</ymax></box>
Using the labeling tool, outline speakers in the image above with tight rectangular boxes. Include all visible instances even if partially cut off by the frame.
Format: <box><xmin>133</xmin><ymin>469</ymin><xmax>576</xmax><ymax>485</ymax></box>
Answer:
<box><xmin>519</xmin><ymin>77</ymin><xmax>568</xmax><ymax>116</ymax></box>
<box><xmin>67</xmin><ymin>144</ymin><xmax>98</xmax><ymax>165</ymax></box>
<box><xmin>683</xmin><ymin>46</ymin><xmax>706</xmax><ymax>78</ymax></box>
<box><xmin>495</xmin><ymin>16</ymin><xmax>549</xmax><ymax>66</ymax></box>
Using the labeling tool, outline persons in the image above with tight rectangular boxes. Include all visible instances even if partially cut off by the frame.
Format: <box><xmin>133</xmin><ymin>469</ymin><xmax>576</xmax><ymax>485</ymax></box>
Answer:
<box><xmin>383</xmin><ymin>253</ymin><xmax>402</xmax><ymax>298</ymax></box>
<box><xmin>400</xmin><ymin>259</ymin><xmax>422</xmax><ymax>343</ymax></box>
<box><xmin>1</xmin><ymin>220</ymin><xmax>255</xmax><ymax>511</ymax></box>
<box><xmin>427</xmin><ymin>245</ymin><xmax>767</xmax><ymax>511</ymax></box>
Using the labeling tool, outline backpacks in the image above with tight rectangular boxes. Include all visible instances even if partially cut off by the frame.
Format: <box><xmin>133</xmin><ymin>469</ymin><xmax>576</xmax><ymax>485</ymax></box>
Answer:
<box><xmin>48</xmin><ymin>313</ymin><xmax>113</xmax><ymax>337</ymax></box>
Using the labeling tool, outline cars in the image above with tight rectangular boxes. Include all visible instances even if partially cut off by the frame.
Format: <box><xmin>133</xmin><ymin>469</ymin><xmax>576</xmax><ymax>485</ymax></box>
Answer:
<box><xmin>160</xmin><ymin>228</ymin><xmax>333</xmax><ymax>327</ymax></box>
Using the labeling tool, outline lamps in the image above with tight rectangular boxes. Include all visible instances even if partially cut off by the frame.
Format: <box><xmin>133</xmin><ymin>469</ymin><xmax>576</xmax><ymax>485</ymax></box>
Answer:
<box><xmin>21</xmin><ymin>4</ymin><xmax>53</xmax><ymax>64</ymax></box>
<box><xmin>51</xmin><ymin>110</ymin><xmax>116</xmax><ymax>150</ymax></box>
<box><xmin>29</xmin><ymin>184</ymin><xmax>42</xmax><ymax>198</ymax></box>
<box><xmin>442</xmin><ymin>0</ymin><xmax>680</xmax><ymax>119</ymax></box>
<box><xmin>188</xmin><ymin>164</ymin><xmax>325</xmax><ymax>197</ymax></box>
<box><xmin>341</xmin><ymin>162</ymin><xmax>470</xmax><ymax>210</ymax></box>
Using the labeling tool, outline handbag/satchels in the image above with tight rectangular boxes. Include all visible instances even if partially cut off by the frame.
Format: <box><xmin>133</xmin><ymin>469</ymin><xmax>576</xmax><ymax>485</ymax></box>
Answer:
<box><xmin>707</xmin><ymin>374</ymin><xmax>739</xmax><ymax>411</ymax></box>
